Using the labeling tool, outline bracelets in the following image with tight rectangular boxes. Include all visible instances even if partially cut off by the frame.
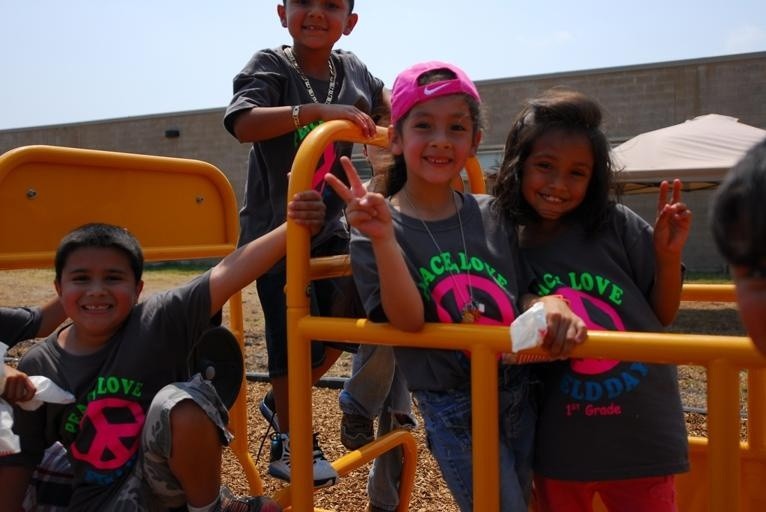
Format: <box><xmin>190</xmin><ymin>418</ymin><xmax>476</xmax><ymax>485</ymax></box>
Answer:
<box><xmin>291</xmin><ymin>104</ymin><xmax>303</xmax><ymax>131</ymax></box>
<box><xmin>283</xmin><ymin>47</ymin><xmax>336</xmax><ymax>104</ymax></box>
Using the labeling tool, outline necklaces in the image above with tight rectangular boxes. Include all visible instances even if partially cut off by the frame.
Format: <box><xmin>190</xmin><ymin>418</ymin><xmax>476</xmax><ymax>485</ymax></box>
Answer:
<box><xmin>399</xmin><ymin>177</ymin><xmax>486</xmax><ymax>324</ymax></box>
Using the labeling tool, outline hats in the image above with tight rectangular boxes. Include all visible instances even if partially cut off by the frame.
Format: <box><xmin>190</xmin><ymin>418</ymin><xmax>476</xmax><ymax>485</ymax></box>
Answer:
<box><xmin>391</xmin><ymin>60</ymin><xmax>482</xmax><ymax>125</ymax></box>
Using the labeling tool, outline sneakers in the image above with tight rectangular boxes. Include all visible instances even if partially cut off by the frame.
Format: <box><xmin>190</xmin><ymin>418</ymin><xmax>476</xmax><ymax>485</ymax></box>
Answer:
<box><xmin>340</xmin><ymin>410</ymin><xmax>375</xmax><ymax>452</ymax></box>
<box><xmin>260</xmin><ymin>388</ymin><xmax>279</xmax><ymax>433</ymax></box>
<box><xmin>268</xmin><ymin>435</ymin><xmax>339</xmax><ymax>489</ymax></box>
<box><xmin>185</xmin><ymin>490</ymin><xmax>281</xmax><ymax>511</ymax></box>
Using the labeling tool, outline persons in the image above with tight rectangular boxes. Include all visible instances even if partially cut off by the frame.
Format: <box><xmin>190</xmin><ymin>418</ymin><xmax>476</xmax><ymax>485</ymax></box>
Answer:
<box><xmin>486</xmin><ymin>89</ymin><xmax>694</xmax><ymax>510</ymax></box>
<box><xmin>220</xmin><ymin>0</ymin><xmax>393</xmax><ymax>487</ymax></box>
<box><xmin>336</xmin><ymin>110</ymin><xmax>415</xmax><ymax>510</ymax></box>
<box><xmin>11</xmin><ymin>188</ymin><xmax>328</xmax><ymax>510</ymax></box>
<box><xmin>324</xmin><ymin>62</ymin><xmax>535</xmax><ymax>510</ymax></box>
<box><xmin>0</xmin><ymin>362</ymin><xmax>36</xmax><ymax>405</ymax></box>
<box><xmin>707</xmin><ymin>136</ymin><xmax>766</xmax><ymax>358</ymax></box>
<box><xmin>1</xmin><ymin>292</ymin><xmax>70</xmax><ymax>349</ymax></box>
